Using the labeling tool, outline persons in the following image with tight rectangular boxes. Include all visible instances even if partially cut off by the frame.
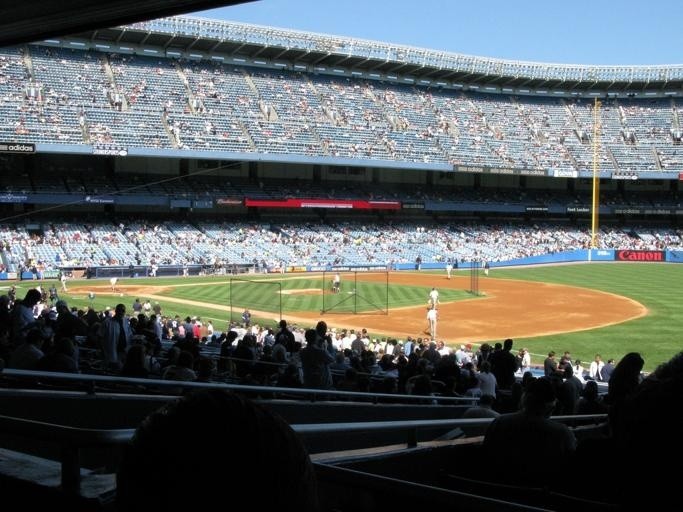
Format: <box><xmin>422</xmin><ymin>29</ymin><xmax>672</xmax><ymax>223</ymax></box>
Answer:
<box><xmin>1</xmin><ymin>284</ymin><xmax>683</xmax><ymax>511</ymax></box>
<box><xmin>2</xmin><ymin>48</ymin><xmax>682</xmax><ymax>294</ymax></box>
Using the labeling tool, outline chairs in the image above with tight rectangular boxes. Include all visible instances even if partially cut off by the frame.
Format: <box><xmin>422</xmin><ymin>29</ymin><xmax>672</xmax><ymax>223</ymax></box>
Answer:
<box><xmin>0</xmin><ymin>42</ymin><xmax>682</xmax><ymax>271</ymax></box>
